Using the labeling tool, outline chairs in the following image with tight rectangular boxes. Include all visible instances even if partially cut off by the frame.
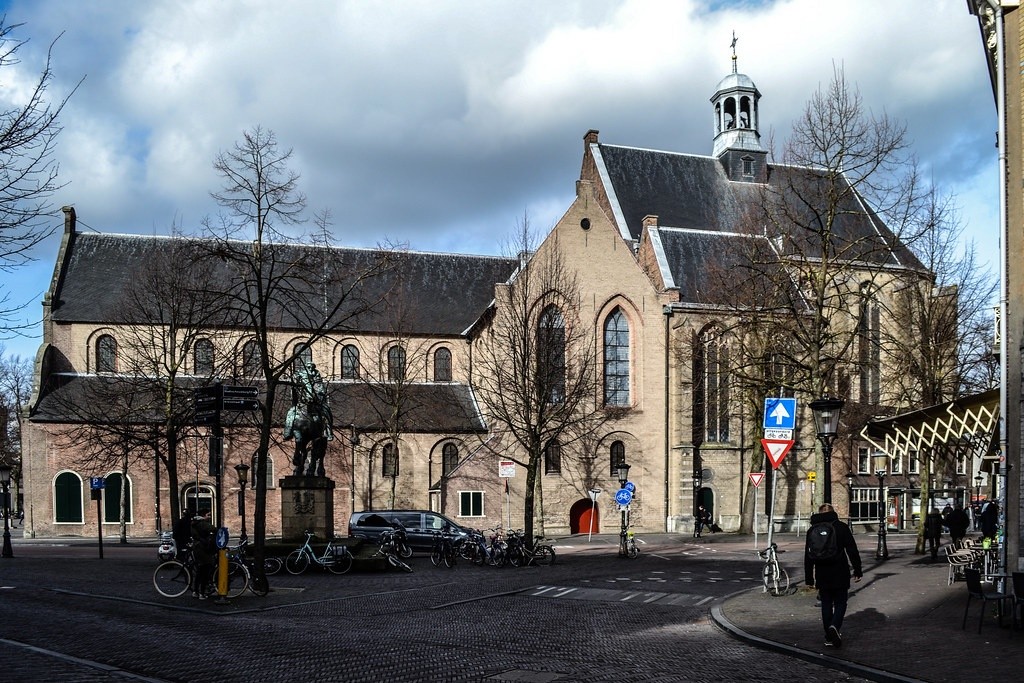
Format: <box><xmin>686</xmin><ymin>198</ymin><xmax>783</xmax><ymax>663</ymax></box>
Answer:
<box><xmin>1011</xmin><ymin>571</ymin><xmax>1024</xmax><ymax>631</ymax></box>
<box><xmin>962</xmin><ymin>567</ymin><xmax>1016</xmax><ymax>635</ymax></box>
<box><xmin>943</xmin><ymin>538</ymin><xmax>999</xmax><ymax>585</ymax></box>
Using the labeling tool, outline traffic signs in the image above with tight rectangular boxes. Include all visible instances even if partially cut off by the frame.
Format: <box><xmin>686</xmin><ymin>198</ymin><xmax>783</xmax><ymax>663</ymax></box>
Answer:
<box><xmin>192</xmin><ymin>386</ymin><xmax>217</xmax><ymax>423</ymax></box>
<box><xmin>224</xmin><ymin>400</ymin><xmax>260</xmax><ymax>412</ymax></box>
<box><xmin>222</xmin><ymin>385</ymin><xmax>262</xmax><ymax>398</ymax></box>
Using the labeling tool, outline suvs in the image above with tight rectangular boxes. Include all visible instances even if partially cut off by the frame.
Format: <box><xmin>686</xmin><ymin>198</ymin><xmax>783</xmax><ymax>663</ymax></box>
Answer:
<box><xmin>347</xmin><ymin>509</ymin><xmax>487</xmax><ymax>559</ymax></box>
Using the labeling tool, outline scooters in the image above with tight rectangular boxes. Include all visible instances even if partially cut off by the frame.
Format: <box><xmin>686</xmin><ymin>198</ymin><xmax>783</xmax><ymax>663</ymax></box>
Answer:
<box><xmin>347</xmin><ymin>517</ymin><xmax>414</xmax><ymax>561</ymax></box>
<box><xmin>155</xmin><ymin>528</ymin><xmax>178</xmax><ymax>565</ymax></box>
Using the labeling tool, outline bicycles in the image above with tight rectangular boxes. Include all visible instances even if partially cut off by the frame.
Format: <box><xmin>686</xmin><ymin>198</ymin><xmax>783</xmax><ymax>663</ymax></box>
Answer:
<box><xmin>368</xmin><ymin>523</ymin><xmax>557</xmax><ymax>574</ymax></box>
<box><xmin>619</xmin><ymin>523</ymin><xmax>638</xmax><ymax>559</ymax></box>
<box><xmin>760</xmin><ymin>542</ymin><xmax>791</xmax><ymax>597</ymax></box>
<box><xmin>285</xmin><ymin>529</ymin><xmax>355</xmax><ymax>576</ymax></box>
<box><xmin>153</xmin><ymin>537</ymin><xmax>271</xmax><ymax>600</ymax></box>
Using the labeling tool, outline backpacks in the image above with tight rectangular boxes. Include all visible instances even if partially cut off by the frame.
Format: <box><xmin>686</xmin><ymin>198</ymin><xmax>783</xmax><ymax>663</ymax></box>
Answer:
<box><xmin>807</xmin><ymin>519</ymin><xmax>844</xmax><ymax>559</ymax></box>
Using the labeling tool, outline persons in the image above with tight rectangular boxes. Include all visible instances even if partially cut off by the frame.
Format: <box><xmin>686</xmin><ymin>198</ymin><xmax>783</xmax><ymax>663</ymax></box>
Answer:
<box><xmin>18</xmin><ymin>510</ymin><xmax>24</xmax><ymax>524</ymax></box>
<box><xmin>698</xmin><ymin>505</ymin><xmax>712</xmax><ymax>532</ymax></box>
<box><xmin>942</xmin><ymin>504</ymin><xmax>953</xmax><ymax>533</ymax></box>
<box><xmin>946</xmin><ymin>503</ymin><xmax>970</xmax><ymax>544</ymax></box>
<box><xmin>924</xmin><ymin>508</ymin><xmax>947</xmax><ymax>558</ymax></box>
<box><xmin>290</xmin><ymin>362</ymin><xmax>331</xmax><ymax>438</ymax></box>
<box><xmin>965</xmin><ymin>500</ymin><xmax>999</xmax><ymax>541</ymax></box>
<box><xmin>174</xmin><ymin>507</ymin><xmax>217</xmax><ymax>599</ymax></box>
<box><xmin>804</xmin><ymin>503</ymin><xmax>862</xmax><ymax>646</ymax></box>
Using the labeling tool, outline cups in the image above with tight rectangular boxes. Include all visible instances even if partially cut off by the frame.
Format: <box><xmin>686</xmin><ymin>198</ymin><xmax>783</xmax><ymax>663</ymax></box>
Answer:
<box><xmin>983</xmin><ymin>541</ymin><xmax>989</xmax><ymax>549</ymax></box>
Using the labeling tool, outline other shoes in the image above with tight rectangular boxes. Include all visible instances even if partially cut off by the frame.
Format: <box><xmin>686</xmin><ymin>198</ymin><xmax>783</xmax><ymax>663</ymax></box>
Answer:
<box><xmin>191</xmin><ymin>592</ymin><xmax>198</xmax><ymax>597</ymax></box>
<box><xmin>829</xmin><ymin>626</ymin><xmax>842</xmax><ymax>648</ymax></box>
<box><xmin>199</xmin><ymin>593</ymin><xmax>208</xmax><ymax>600</ymax></box>
<box><xmin>823</xmin><ymin>638</ymin><xmax>833</xmax><ymax>647</ymax></box>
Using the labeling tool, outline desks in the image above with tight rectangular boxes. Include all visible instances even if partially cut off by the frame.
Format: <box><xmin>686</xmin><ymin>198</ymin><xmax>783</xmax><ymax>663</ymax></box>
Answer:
<box><xmin>983</xmin><ymin>573</ymin><xmax>1015</xmax><ymax>618</ymax></box>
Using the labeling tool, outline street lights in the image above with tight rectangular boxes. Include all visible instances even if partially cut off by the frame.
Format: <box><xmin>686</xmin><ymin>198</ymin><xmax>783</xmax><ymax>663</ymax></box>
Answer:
<box><xmin>974</xmin><ymin>475</ymin><xmax>985</xmax><ymax>531</ymax></box>
<box><xmin>807</xmin><ymin>397</ymin><xmax>848</xmax><ymax>504</ymax></box>
<box><xmin>0</xmin><ymin>462</ymin><xmax>14</xmax><ymax>559</ymax></box>
<box><xmin>614</xmin><ymin>458</ymin><xmax>631</xmax><ymax>559</ymax></box>
<box><xmin>691</xmin><ymin>471</ymin><xmax>704</xmax><ymax>538</ymax></box>
<box><xmin>870</xmin><ymin>449</ymin><xmax>890</xmax><ymax>560</ymax></box>
<box><xmin>233</xmin><ymin>460</ymin><xmax>250</xmax><ymax>554</ymax></box>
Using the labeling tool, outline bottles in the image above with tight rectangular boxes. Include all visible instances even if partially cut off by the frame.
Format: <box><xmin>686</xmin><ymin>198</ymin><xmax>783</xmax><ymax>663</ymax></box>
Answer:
<box><xmin>995</xmin><ymin>532</ymin><xmax>1000</xmax><ymax>544</ymax></box>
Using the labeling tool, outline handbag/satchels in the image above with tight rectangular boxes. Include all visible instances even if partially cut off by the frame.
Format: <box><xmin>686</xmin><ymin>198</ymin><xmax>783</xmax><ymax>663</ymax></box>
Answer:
<box><xmin>923</xmin><ymin>522</ymin><xmax>930</xmax><ymax>539</ymax></box>
